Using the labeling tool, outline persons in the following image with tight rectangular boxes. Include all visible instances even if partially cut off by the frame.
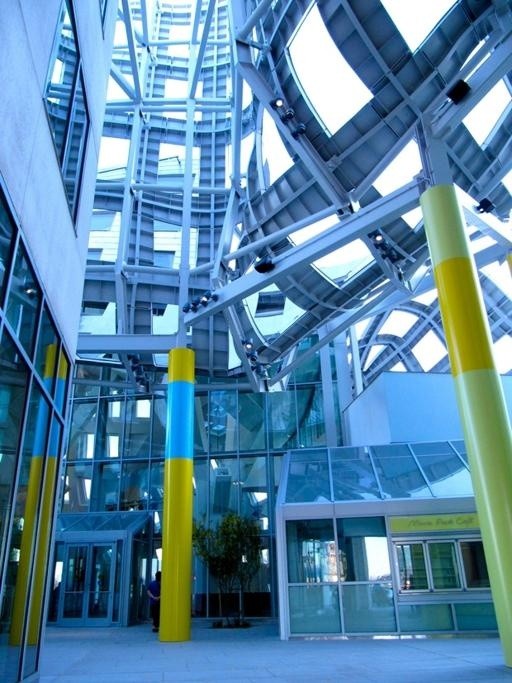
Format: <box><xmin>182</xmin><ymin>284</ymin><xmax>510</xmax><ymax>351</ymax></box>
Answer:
<box><xmin>72</xmin><ymin>575</ymin><xmax>80</xmax><ymax>610</ymax></box>
<box><xmin>404</xmin><ymin>580</ymin><xmax>414</xmax><ymax>590</ymax></box>
<box><xmin>54</xmin><ymin>581</ymin><xmax>61</xmax><ymax>618</ymax></box>
<box><xmin>145</xmin><ymin>570</ymin><xmax>161</xmax><ymax>633</ymax></box>
<box><xmin>95</xmin><ymin>575</ymin><xmax>106</xmax><ymax>609</ymax></box>
